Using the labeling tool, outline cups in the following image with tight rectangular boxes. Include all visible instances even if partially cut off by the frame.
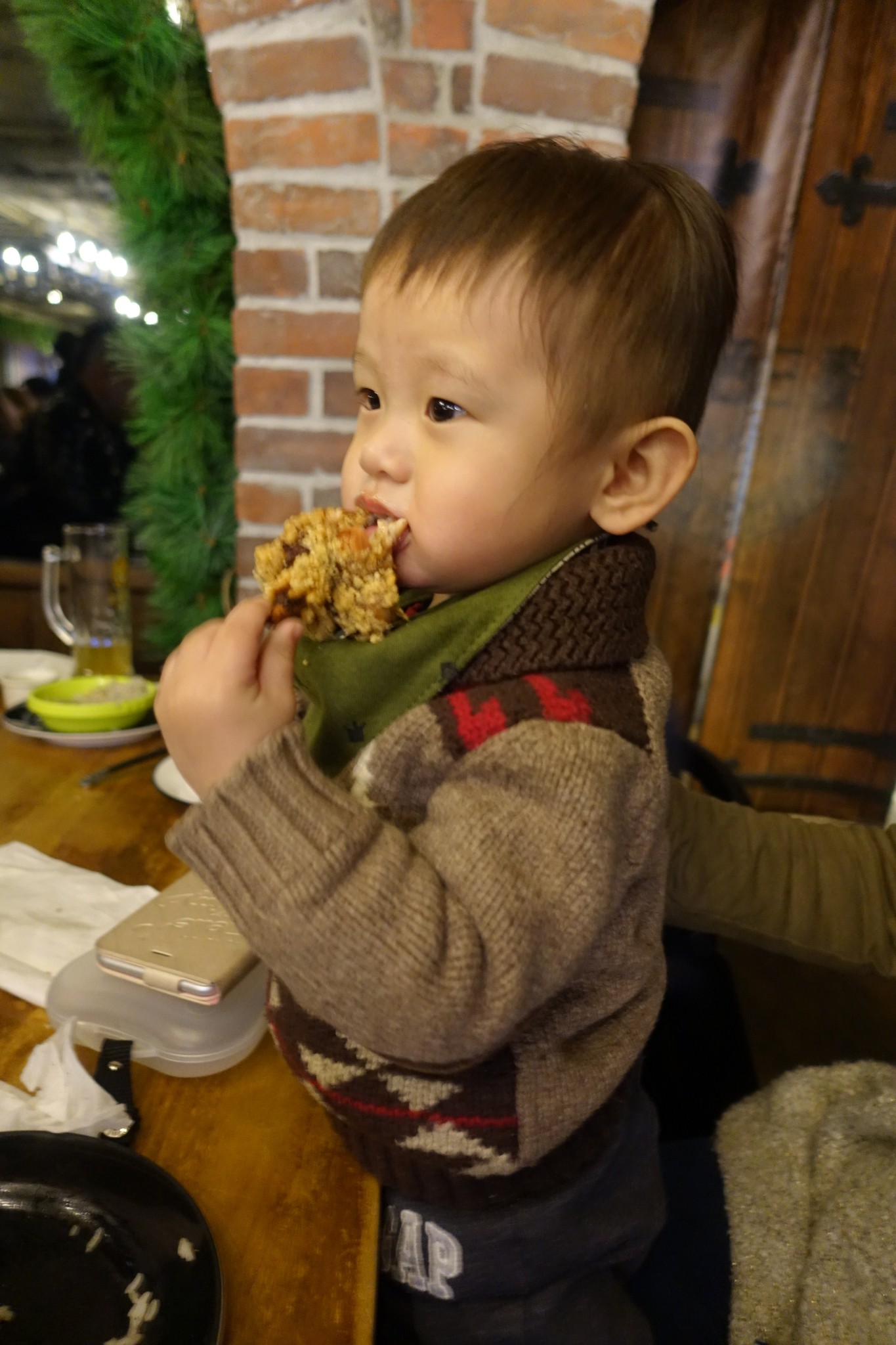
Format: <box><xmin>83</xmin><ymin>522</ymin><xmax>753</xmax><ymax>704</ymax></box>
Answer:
<box><xmin>40</xmin><ymin>523</ymin><xmax>134</xmax><ymax>676</ymax></box>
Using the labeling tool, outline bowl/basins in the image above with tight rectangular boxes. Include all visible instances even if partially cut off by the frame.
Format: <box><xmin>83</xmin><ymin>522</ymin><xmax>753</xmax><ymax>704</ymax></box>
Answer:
<box><xmin>24</xmin><ymin>674</ymin><xmax>158</xmax><ymax>733</ymax></box>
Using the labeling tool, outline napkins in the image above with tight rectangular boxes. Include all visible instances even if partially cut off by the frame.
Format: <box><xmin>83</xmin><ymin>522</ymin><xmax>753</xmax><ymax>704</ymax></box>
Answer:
<box><xmin>0</xmin><ymin>839</ymin><xmax>162</xmax><ymax>1009</ymax></box>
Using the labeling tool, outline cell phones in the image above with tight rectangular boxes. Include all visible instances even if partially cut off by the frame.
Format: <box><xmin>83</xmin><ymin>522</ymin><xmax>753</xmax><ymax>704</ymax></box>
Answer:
<box><xmin>93</xmin><ymin>870</ymin><xmax>263</xmax><ymax>1006</ymax></box>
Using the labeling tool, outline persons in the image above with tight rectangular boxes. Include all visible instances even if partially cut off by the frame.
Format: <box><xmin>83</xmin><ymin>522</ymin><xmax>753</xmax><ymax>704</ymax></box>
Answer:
<box><xmin>156</xmin><ymin>138</ymin><xmax>738</xmax><ymax>1345</ymax></box>
<box><xmin>1</xmin><ymin>323</ymin><xmax>139</xmax><ymax>565</ymax></box>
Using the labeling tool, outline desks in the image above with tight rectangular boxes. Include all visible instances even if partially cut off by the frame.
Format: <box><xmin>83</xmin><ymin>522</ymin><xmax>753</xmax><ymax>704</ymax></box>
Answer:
<box><xmin>0</xmin><ymin>667</ymin><xmax>377</xmax><ymax>1345</ymax></box>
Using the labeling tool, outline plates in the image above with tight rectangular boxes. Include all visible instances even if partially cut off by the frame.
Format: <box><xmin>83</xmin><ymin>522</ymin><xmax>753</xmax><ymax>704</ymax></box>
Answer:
<box><xmin>152</xmin><ymin>755</ymin><xmax>203</xmax><ymax>805</ymax></box>
<box><xmin>0</xmin><ymin>1130</ymin><xmax>225</xmax><ymax>1345</ymax></box>
<box><xmin>0</xmin><ymin>646</ymin><xmax>72</xmax><ymax>713</ymax></box>
<box><xmin>1</xmin><ymin>700</ymin><xmax>160</xmax><ymax>749</ymax></box>
